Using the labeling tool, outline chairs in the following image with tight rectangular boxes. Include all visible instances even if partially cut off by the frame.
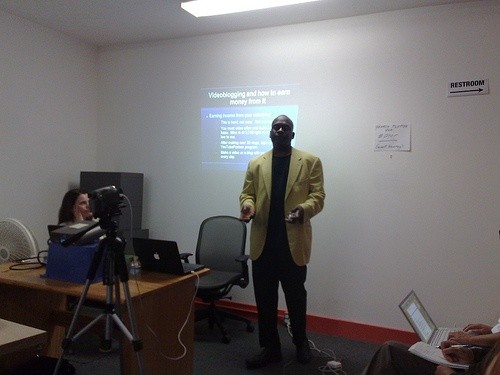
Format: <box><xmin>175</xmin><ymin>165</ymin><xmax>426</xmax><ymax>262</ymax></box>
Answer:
<box><xmin>180</xmin><ymin>215</ymin><xmax>255</xmax><ymax>346</ymax></box>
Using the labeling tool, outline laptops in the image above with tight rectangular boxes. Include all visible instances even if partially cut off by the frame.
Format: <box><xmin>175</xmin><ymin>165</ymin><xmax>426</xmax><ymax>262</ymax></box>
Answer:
<box><xmin>398</xmin><ymin>289</ymin><xmax>484</xmax><ymax>350</ymax></box>
<box><xmin>133</xmin><ymin>236</ymin><xmax>204</xmax><ymax>276</ymax></box>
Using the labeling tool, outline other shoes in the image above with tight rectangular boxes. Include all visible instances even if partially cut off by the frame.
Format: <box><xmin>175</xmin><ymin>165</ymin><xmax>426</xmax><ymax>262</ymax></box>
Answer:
<box><xmin>245</xmin><ymin>343</ymin><xmax>283</xmax><ymax>368</ymax></box>
<box><xmin>293</xmin><ymin>336</ymin><xmax>312</xmax><ymax>364</ymax></box>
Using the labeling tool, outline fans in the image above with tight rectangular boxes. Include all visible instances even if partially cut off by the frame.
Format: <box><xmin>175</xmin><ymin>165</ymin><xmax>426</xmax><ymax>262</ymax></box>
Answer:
<box><xmin>0</xmin><ymin>217</ymin><xmax>42</xmax><ymax>265</ymax></box>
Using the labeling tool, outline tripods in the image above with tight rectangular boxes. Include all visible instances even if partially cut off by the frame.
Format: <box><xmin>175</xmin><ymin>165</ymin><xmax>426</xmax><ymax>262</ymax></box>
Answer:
<box><xmin>55</xmin><ymin>228</ymin><xmax>144</xmax><ymax>375</ymax></box>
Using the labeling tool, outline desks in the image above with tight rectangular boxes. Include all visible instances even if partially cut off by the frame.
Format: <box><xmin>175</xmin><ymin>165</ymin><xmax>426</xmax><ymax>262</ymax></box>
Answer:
<box><xmin>0</xmin><ymin>319</ymin><xmax>48</xmax><ymax>375</ymax></box>
<box><xmin>0</xmin><ymin>260</ymin><xmax>210</xmax><ymax>375</ymax></box>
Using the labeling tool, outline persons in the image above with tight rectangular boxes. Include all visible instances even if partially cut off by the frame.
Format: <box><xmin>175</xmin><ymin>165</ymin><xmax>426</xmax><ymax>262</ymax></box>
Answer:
<box><xmin>58</xmin><ymin>188</ymin><xmax>93</xmax><ymax>226</ymax></box>
<box><xmin>240</xmin><ymin>114</ymin><xmax>325</xmax><ymax>369</ymax></box>
<box><xmin>362</xmin><ymin>319</ymin><xmax>500</xmax><ymax>375</ymax></box>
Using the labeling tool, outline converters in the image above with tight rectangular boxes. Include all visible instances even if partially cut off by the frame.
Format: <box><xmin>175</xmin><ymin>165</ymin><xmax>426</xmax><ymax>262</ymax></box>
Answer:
<box><xmin>328</xmin><ymin>360</ymin><xmax>342</xmax><ymax>370</ymax></box>
